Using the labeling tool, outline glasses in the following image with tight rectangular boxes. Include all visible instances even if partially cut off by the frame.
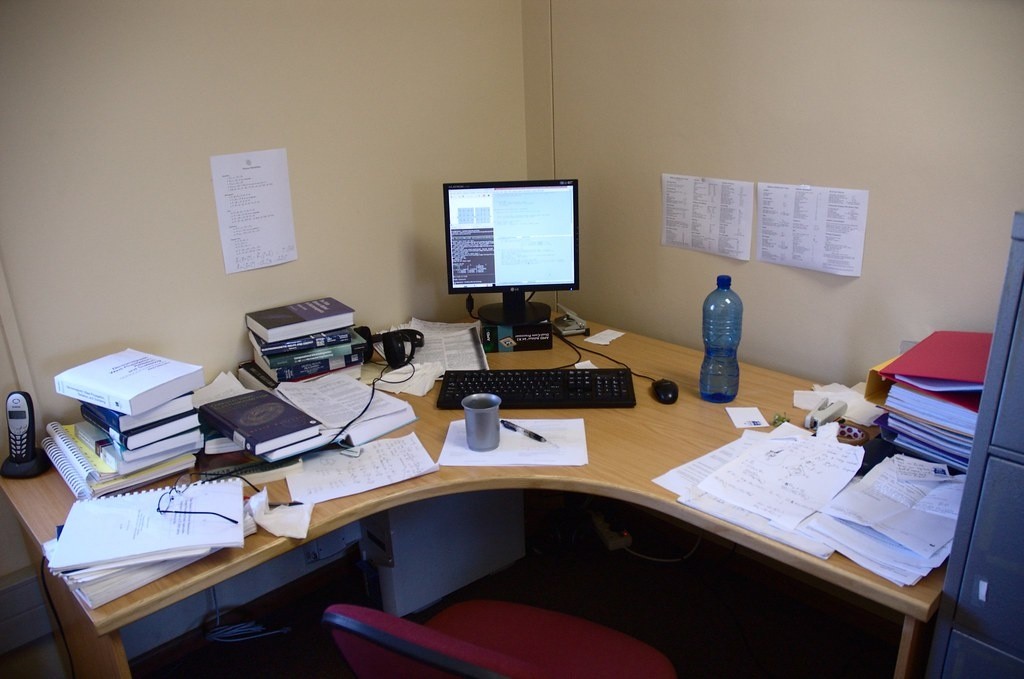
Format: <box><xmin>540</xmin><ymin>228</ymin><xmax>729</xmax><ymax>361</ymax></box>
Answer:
<box><xmin>156</xmin><ymin>472</ymin><xmax>260</xmax><ymax>524</ymax></box>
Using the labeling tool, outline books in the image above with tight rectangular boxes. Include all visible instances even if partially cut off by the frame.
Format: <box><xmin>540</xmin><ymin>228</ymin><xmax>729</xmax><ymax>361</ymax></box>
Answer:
<box><xmin>41</xmin><ymin>348</ymin><xmax>206</xmax><ymax>499</ymax></box>
<box><xmin>258</xmin><ymin>371</ymin><xmax>420</xmax><ymax>463</ymax></box>
<box><xmin>864</xmin><ymin>331</ymin><xmax>994</xmax><ymax>473</ymax></box>
<box><xmin>43</xmin><ymin>479</ymin><xmax>258</xmax><ymax>609</ymax></box>
<box><xmin>236</xmin><ymin>297</ymin><xmax>367</xmax><ymax>395</ymax></box>
<box><xmin>198</xmin><ymin>389</ymin><xmax>323</xmax><ymax>456</ymax></box>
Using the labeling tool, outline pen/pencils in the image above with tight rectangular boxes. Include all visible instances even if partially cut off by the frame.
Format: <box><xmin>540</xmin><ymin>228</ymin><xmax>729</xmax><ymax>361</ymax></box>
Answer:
<box><xmin>500</xmin><ymin>419</ymin><xmax>547</xmax><ymax>444</ymax></box>
<box><xmin>268</xmin><ymin>501</ymin><xmax>304</xmax><ymax>506</ymax></box>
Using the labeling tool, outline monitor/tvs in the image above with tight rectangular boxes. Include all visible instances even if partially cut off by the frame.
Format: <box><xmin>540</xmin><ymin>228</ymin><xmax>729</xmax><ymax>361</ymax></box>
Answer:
<box><xmin>443</xmin><ymin>179</ymin><xmax>579</xmax><ymax>325</ymax></box>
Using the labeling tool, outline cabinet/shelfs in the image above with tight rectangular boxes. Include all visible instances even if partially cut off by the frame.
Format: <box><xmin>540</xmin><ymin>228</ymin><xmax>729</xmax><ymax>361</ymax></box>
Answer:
<box><xmin>924</xmin><ymin>210</ymin><xmax>1024</xmax><ymax>679</ymax></box>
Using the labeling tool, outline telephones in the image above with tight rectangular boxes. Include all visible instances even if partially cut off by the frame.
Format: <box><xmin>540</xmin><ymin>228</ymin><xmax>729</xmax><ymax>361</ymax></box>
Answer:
<box><xmin>0</xmin><ymin>389</ymin><xmax>46</xmax><ymax>479</ymax></box>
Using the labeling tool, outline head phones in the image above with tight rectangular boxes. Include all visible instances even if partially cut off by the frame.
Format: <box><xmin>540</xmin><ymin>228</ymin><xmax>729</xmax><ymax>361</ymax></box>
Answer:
<box><xmin>353</xmin><ymin>326</ymin><xmax>425</xmax><ymax>369</ymax></box>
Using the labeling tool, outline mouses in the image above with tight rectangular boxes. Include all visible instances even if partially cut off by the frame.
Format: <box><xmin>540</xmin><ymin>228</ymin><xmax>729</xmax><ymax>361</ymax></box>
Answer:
<box><xmin>651</xmin><ymin>378</ymin><xmax>679</xmax><ymax>404</ymax></box>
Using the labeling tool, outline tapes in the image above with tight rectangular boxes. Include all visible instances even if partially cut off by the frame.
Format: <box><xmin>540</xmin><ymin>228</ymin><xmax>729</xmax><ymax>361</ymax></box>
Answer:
<box><xmin>836</xmin><ymin>424</ymin><xmax>870</xmax><ymax>444</ymax></box>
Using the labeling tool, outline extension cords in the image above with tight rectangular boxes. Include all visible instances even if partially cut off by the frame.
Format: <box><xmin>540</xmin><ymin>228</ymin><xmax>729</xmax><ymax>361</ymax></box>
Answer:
<box><xmin>586</xmin><ymin>510</ymin><xmax>632</xmax><ymax>551</ymax></box>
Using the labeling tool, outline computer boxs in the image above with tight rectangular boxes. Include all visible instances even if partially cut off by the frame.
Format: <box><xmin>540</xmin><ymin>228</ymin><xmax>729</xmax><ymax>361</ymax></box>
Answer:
<box><xmin>358</xmin><ymin>490</ymin><xmax>528</xmax><ymax>620</ymax></box>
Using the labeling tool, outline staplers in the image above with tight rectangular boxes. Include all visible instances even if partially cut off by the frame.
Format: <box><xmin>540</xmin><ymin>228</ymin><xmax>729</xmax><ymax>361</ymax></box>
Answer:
<box><xmin>804</xmin><ymin>398</ymin><xmax>848</xmax><ymax>430</ymax></box>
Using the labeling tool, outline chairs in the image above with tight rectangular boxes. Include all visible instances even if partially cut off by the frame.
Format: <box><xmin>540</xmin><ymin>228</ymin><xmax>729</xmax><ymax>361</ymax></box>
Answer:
<box><xmin>322</xmin><ymin>599</ymin><xmax>678</xmax><ymax>679</ymax></box>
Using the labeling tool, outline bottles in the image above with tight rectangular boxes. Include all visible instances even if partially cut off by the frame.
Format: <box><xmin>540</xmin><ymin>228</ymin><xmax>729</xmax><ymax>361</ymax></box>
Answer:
<box><xmin>700</xmin><ymin>275</ymin><xmax>743</xmax><ymax>402</ymax></box>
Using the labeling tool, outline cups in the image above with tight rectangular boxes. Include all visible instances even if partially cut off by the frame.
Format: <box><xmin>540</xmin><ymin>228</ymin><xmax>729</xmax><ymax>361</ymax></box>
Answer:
<box><xmin>461</xmin><ymin>393</ymin><xmax>501</xmax><ymax>451</ymax></box>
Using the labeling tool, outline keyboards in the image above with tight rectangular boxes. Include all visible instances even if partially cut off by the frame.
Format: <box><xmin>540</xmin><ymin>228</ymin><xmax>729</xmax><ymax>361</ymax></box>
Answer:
<box><xmin>437</xmin><ymin>367</ymin><xmax>636</xmax><ymax>408</ymax></box>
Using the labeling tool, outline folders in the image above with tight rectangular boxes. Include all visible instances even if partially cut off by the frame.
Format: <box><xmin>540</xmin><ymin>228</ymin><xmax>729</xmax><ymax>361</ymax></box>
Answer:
<box><xmin>863</xmin><ymin>330</ymin><xmax>994</xmax><ymax>474</ymax></box>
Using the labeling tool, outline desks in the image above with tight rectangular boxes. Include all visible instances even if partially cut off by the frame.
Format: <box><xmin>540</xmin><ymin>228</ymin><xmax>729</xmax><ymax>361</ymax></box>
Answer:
<box><xmin>0</xmin><ymin>311</ymin><xmax>947</xmax><ymax>679</ymax></box>
